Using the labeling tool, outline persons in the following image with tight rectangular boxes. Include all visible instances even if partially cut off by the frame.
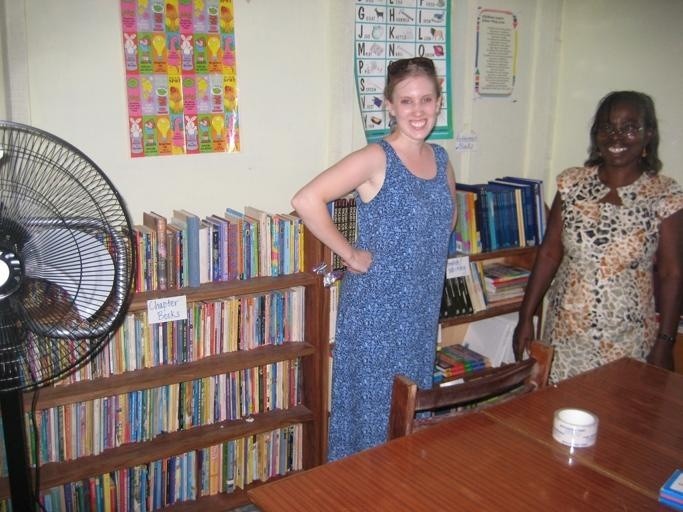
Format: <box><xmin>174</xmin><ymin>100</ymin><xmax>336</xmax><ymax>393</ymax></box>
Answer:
<box><xmin>291</xmin><ymin>57</ymin><xmax>458</xmax><ymax>463</ymax></box>
<box><xmin>512</xmin><ymin>90</ymin><xmax>682</xmax><ymax>386</ymax></box>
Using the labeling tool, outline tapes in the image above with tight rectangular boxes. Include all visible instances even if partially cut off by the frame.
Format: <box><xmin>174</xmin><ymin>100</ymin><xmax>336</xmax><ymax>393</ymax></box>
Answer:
<box><xmin>552</xmin><ymin>406</ymin><xmax>600</xmax><ymax>448</ymax></box>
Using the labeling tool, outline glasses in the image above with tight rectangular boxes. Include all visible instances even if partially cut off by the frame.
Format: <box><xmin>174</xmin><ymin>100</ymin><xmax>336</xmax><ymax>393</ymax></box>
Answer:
<box><xmin>388</xmin><ymin>58</ymin><xmax>433</xmax><ymax>84</ymax></box>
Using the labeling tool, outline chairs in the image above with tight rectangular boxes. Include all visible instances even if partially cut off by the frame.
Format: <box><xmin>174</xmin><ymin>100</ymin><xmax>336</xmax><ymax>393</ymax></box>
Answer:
<box><xmin>387</xmin><ymin>341</ymin><xmax>554</xmax><ymax>445</ymax></box>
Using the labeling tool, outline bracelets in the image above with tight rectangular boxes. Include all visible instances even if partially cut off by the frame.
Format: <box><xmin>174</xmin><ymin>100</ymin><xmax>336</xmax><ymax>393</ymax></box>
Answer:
<box><xmin>658</xmin><ymin>333</ymin><xmax>678</xmax><ymax>343</ymax></box>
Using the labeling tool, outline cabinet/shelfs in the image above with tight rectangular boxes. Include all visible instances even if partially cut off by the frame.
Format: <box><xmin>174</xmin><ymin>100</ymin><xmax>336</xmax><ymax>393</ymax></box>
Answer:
<box><xmin>320</xmin><ymin>200</ymin><xmax>551</xmax><ymax>464</ymax></box>
<box><xmin>0</xmin><ymin>210</ymin><xmax>322</xmax><ymax>512</ymax></box>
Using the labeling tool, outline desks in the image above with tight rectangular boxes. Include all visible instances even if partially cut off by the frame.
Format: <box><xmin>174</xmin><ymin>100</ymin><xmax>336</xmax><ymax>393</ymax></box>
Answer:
<box><xmin>245</xmin><ymin>355</ymin><xmax>683</xmax><ymax>512</ymax></box>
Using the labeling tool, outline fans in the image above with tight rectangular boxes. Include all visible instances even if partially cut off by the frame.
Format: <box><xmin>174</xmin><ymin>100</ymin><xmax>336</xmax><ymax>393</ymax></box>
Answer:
<box><xmin>1</xmin><ymin>114</ymin><xmax>141</xmax><ymax>512</ymax></box>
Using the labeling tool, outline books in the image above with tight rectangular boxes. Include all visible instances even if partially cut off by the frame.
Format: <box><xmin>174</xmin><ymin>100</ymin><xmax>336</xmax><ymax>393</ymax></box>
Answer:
<box><xmin>327</xmin><ymin>177</ymin><xmax>546</xmax><ymax>412</ymax></box>
<box><xmin>658</xmin><ymin>470</ymin><xmax>683</xmax><ymax>512</ymax></box>
<box><xmin>19</xmin><ymin>207</ymin><xmax>307</xmax><ymax>512</ymax></box>
